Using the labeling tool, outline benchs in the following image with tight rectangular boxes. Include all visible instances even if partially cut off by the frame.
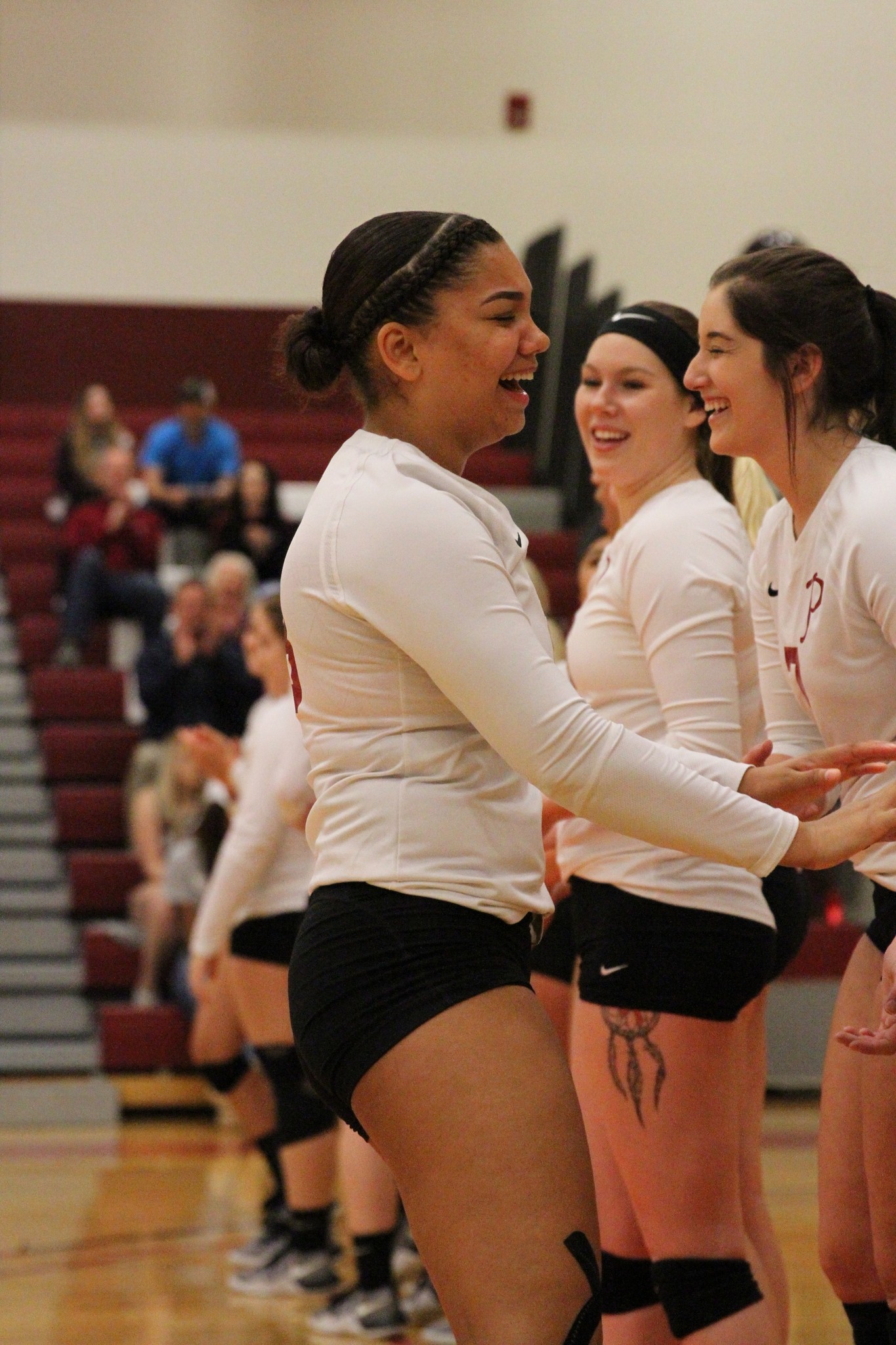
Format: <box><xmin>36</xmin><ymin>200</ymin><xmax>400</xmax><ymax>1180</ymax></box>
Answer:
<box><xmin>0</xmin><ymin>401</ymin><xmax>869</xmax><ymax>1128</ymax></box>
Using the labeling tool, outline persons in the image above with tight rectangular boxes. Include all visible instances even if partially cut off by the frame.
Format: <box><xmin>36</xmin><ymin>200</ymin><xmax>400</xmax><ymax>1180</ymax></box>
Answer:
<box><xmin>276</xmin><ymin>210</ymin><xmax>896</xmax><ymax>1345</ymax></box>
<box><xmin>51</xmin><ymin>228</ymin><xmax>895</xmax><ymax>1345</ymax></box>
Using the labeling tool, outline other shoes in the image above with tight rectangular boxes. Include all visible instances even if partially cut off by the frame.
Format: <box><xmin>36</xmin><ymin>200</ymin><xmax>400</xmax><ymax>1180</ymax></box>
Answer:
<box><xmin>422</xmin><ymin>1314</ymin><xmax>456</xmax><ymax>1345</ymax></box>
<box><xmin>227</xmin><ymin>1242</ymin><xmax>340</xmax><ymax>1297</ymax></box>
<box><xmin>231</xmin><ymin>1226</ymin><xmax>290</xmax><ymax>1269</ymax></box>
<box><xmin>305</xmin><ymin>1284</ymin><xmax>398</xmax><ymax>1335</ymax></box>
<box><xmin>345</xmin><ymin>1280</ymin><xmax>443</xmax><ymax>1338</ymax></box>
